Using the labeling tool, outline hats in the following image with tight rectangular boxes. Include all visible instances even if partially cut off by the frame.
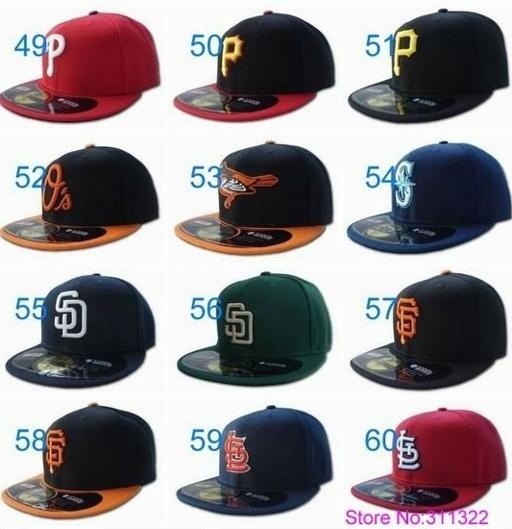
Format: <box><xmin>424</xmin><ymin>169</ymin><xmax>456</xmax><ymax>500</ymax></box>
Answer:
<box><xmin>0</xmin><ymin>9</ymin><xmax>160</xmax><ymax>126</ymax></box>
<box><xmin>177</xmin><ymin>270</ymin><xmax>332</xmax><ymax>388</ymax></box>
<box><xmin>349</xmin><ymin>270</ymin><xmax>511</xmax><ymax>392</ymax></box>
<box><xmin>169</xmin><ymin>138</ymin><xmax>337</xmax><ymax>257</ymax></box>
<box><xmin>175</xmin><ymin>403</ymin><xmax>336</xmax><ymax>519</ymax></box>
<box><xmin>168</xmin><ymin>8</ymin><xmax>336</xmax><ymax>124</ymax></box>
<box><xmin>0</xmin><ymin>142</ymin><xmax>160</xmax><ymax>253</ymax></box>
<box><xmin>4</xmin><ymin>273</ymin><xmax>160</xmax><ymax>390</ymax></box>
<box><xmin>343</xmin><ymin>8</ymin><xmax>512</xmax><ymax>124</ymax></box>
<box><xmin>346</xmin><ymin>405</ymin><xmax>511</xmax><ymax>519</ymax></box>
<box><xmin>344</xmin><ymin>138</ymin><xmax>512</xmax><ymax>257</ymax></box>
<box><xmin>0</xmin><ymin>402</ymin><xmax>156</xmax><ymax>520</ymax></box>
